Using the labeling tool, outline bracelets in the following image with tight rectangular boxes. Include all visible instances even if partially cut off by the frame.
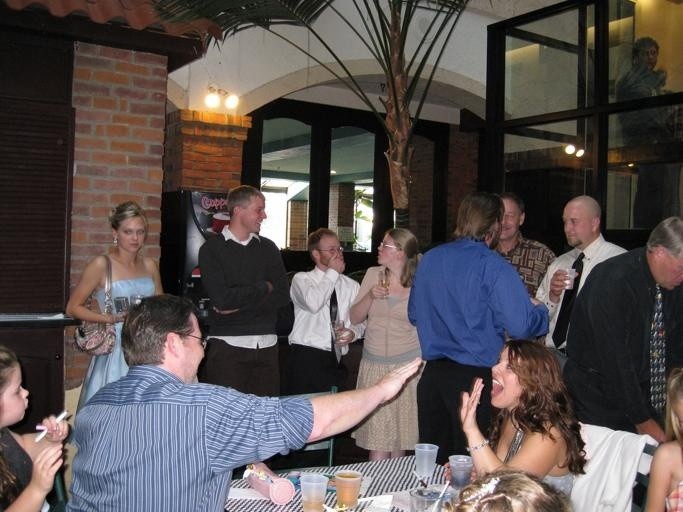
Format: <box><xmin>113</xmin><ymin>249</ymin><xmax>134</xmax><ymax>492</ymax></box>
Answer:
<box><xmin>464</xmin><ymin>439</ymin><xmax>491</xmax><ymax>453</ymax></box>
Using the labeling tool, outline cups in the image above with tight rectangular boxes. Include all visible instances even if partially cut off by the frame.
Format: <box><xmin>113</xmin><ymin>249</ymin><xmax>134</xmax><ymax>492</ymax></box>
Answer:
<box><xmin>409</xmin><ymin>489</ymin><xmax>446</xmax><ymax>512</ymax></box>
<box><xmin>450</xmin><ymin>455</ymin><xmax>472</xmax><ymax>490</ymax></box>
<box><xmin>414</xmin><ymin>444</ymin><xmax>437</xmax><ymax>477</ymax></box>
<box><xmin>329</xmin><ymin>320</ymin><xmax>344</xmax><ymax>341</ymax></box>
<box><xmin>300</xmin><ymin>475</ymin><xmax>328</xmax><ymax>512</ymax></box>
<box><xmin>334</xmin><ymin>470</ymin><xmax>361</xmax><ymax>508</ymax></box>
<box><xmin>378</xmin><ymin>270</ymin><xmax>391</xmax><ymax>299</ymax></box>
<box><xmin>130</xmin><ymin>294</ymin><xmax>143</xmax><ymax>305</ymax></box>
<box><xmin>560</xmin><ymin>268</ymin><xmax>575</xmax><ymax>288</ymax></box>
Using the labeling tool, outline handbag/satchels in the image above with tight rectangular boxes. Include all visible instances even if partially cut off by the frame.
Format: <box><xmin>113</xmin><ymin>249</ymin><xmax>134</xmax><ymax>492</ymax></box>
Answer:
<box><xmin>75</xmin><ymin>255</ymin><xmax>116</xmax><ymax>356</ymax></box>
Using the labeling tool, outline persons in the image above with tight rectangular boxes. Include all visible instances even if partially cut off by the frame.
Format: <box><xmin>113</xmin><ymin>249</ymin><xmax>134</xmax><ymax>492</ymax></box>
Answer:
<box><xmin>287</xmin><ymin>227</ymin><xmax>368</xmax><ymax>395</ymax></box>
<box><xmin>562</xmin><ymin>216</ymin><xmax>683</xmax><ymax>444</ymax></box>
<box><xmin>493</xmin><ymin>192</ymin><xmax>557</xmax><ymax>299</ymax></box>
<box><xmin>66</xmin><ymin>294</ymin><xmax>423</xmax><ymax>511</ymax></box>
<box><xmin>406</xmin><ymin>191</ymin><xmax>549</xmax><ymax>466</ymax></box>
<box><xmin>348</xmin><ymin>228</ymin><xmax>425</xmax><ymax>461</ymax></box>
<box><xmin>535</xmin><ymin>194</ymin><xmax>628</xmax><ymax>355</ymax></box>
<box><xmin>197</xmin><ymin>185</ymin><xmax>290</xmax><ymax>397</ymax></box>
<box><xmin>0</xmin><ymin>344</ymin><xmax>70</xmax><ymax>512</ymax></box>
<box><xmin>458</xmin><ymin>338</ymin><xmax>586</xmax><ymax>500</ymax></box>
<box><xmin>645</xmin><ymin>367</ymin><xmax>683</xmax><ymax>511</ymax></box>
<box><xmin>615</xmin><ymin>37</ymin><xmax>674</xmax><ymax>146</ymax></box>
<box><xmin>439</xmin><ymin>469</ymin><xmax>572</xmax><ymax>511</ymax></box>
<box><xmin>66</xmin><ymin>202</ymin><xmax>164</xmax><ymax>418</ymax></box>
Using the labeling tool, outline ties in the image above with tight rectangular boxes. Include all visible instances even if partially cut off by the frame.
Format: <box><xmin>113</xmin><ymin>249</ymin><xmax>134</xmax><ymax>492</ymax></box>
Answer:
<box><xmin>329</xmin><ymin>287</ymin><xmax>341</xmax><ymax>367</ymax></box>
<box><xmin>649</xmin><ymin>283</ymin><xmax>667</xmax><ymax>415</ymax></box>
<box><xmin>552</xmin><ymin>253</ymin><xmax>585</xmax><ymax>349</ymax></box>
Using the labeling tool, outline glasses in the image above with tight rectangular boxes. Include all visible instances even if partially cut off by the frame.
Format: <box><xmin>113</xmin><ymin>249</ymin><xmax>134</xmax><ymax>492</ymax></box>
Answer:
<box><xmin>379</xmin><ymin>241</ymin><xmax>397</xmax><ymax>249</ymax></box>
<box><xmin>176</xmin><ymin>331</ymin><xmax>207</xmax><ymax>350</ymax></box>
<box><xmin>318</xmin><ymin>247</ymin><xmax>343</xmax><ymax>254</ymax></box>
